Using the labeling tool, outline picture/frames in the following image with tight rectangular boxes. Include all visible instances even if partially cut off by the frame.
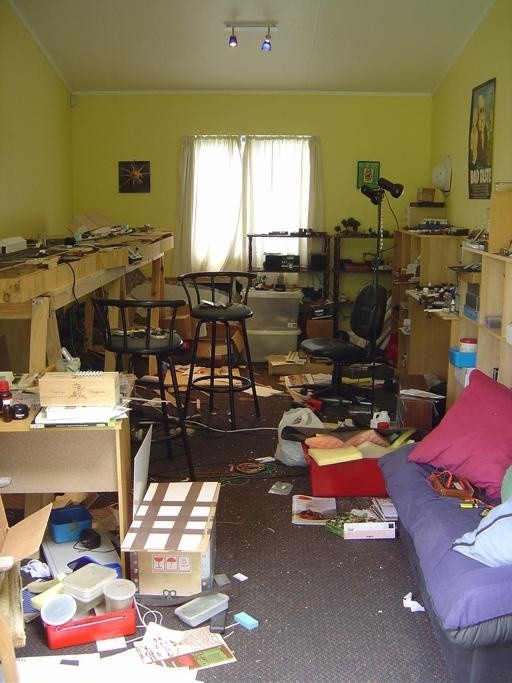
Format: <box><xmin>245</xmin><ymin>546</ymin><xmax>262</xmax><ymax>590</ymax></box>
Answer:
<box><xmin>118</xmin><ymin>160</ymin><xmax>151</xmax><ymax>193</ymax></box>
<box><xmin>358</xmin><ymin>161</ymin><xmax>380</xmax><ymax>188</ymax></box>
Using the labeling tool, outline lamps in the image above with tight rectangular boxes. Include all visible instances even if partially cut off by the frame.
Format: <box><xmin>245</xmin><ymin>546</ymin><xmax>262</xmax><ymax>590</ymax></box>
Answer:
<box><xmin>224</xmin><ymin>20</ymin><xmax>279</xmax><ymax>52</ymax></box>
<box><xmin>352</xmin><ymin>177</ymin><xmax>406</xmax><ymax>428</ymax></box>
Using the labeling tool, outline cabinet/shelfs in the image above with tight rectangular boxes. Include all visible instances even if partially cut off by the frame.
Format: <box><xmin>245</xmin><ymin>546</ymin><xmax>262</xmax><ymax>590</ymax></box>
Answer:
<box><xmin>444</xmin><ymin>245</ymin><xmax>512</xmax><ymax>413</ymax></box>
<box><xmin>334</xmin><ymin>231</ymin><xmax>393</xmax><ymax>337</ymax></box>
<box><xmin>391</xmin><ymin>229</ymin><xmax>469</xmax><ymax>378</ymax></box>
<box><xmin>247</xmin><ymin>233</ymin><xmax>331</xmax><ymax>337</ymax></box>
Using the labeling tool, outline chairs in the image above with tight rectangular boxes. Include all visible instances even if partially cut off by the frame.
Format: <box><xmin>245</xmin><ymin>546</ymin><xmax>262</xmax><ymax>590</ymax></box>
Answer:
<box><xmin>92</xmin><ymin>297</ymin><xmax>195</xmax><ymax>482</ymax></box>
<box><xmin>178</xmin><ymin>273</ymin><xmax>258</xmax><ymax>429</ymax></box>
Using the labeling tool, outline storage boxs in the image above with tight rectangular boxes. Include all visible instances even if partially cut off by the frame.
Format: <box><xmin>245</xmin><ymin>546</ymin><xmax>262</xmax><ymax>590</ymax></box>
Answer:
<box><xmin>130</xmin><ymin>510</ymin><xmax>216</xmax><ymax>592</ymax></box>
<box><xmin>0</xmin><ymin>495</ymin><xmax>52</xmax><ymax>683</ymax></box>
<box><xmin>164</xmin><ymin>288</ymin><xmax>334</xmax><ymax>365</ymax></box>
<box><xmin>417</xmin><ymin>187</ymin><xmax>435</xmax><ymax>202</ymax></box>
<box><xmin>301</xmin><ymin>442</ymin><xmax>389</xmax><ymax>496</ymax></box>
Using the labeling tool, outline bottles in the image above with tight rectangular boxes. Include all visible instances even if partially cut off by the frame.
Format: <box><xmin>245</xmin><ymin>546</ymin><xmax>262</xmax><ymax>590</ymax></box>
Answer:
<box><xmin>0</xmin><ymin>380</ymin><xmax>13</xmax><ymax>423</ymax></box>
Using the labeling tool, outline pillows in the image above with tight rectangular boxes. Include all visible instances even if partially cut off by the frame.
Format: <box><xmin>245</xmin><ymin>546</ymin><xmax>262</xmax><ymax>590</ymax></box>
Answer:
<box><xmin>409</xmin><ymin>368</ymin><xmax>512</xmax><ymax>498</ymax></box>
<box><xmin>453</xmin><ymin>493</ymin><xmax>512</xmax><ymax>566</ymax></box>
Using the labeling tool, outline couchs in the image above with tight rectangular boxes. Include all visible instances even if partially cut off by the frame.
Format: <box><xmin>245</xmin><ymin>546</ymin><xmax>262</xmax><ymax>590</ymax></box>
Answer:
<box><xmin>379</xmin><ymin>372</ymin><xmax>512</xmax><ymax>683</ymax></box>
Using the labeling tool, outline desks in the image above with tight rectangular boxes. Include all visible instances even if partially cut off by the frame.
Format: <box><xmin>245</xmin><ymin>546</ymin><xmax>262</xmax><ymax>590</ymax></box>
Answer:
<box><xmin>0</xmin><ymin>404</ymin><xmax>133</xmax><ymax>580</ymax></box>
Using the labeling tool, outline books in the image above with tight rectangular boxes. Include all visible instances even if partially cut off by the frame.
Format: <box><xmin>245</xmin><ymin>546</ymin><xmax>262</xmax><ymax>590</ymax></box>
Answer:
<box><xmin>29</xmin><ymin>391</ymin><xmax>133</xmax><ymax>428</ymax></box>
<box><xmin>284</xmin><ymin>373</ymin><xmax>315</xmax><ymax>388</ymax></box>
<box><xmin>290</xmin><ymin>493</ymin><xmax>337</xmax><ymax>525</ymax></box>
<box><xmin>369</xmin><ymin>494</ymin><xmax>399</xmax><ymax>529</ymax></box>
<box><xmin>133</xmin><ymin>624</ymin><xmax>238</xmax><ymax>672</ymax></box>
